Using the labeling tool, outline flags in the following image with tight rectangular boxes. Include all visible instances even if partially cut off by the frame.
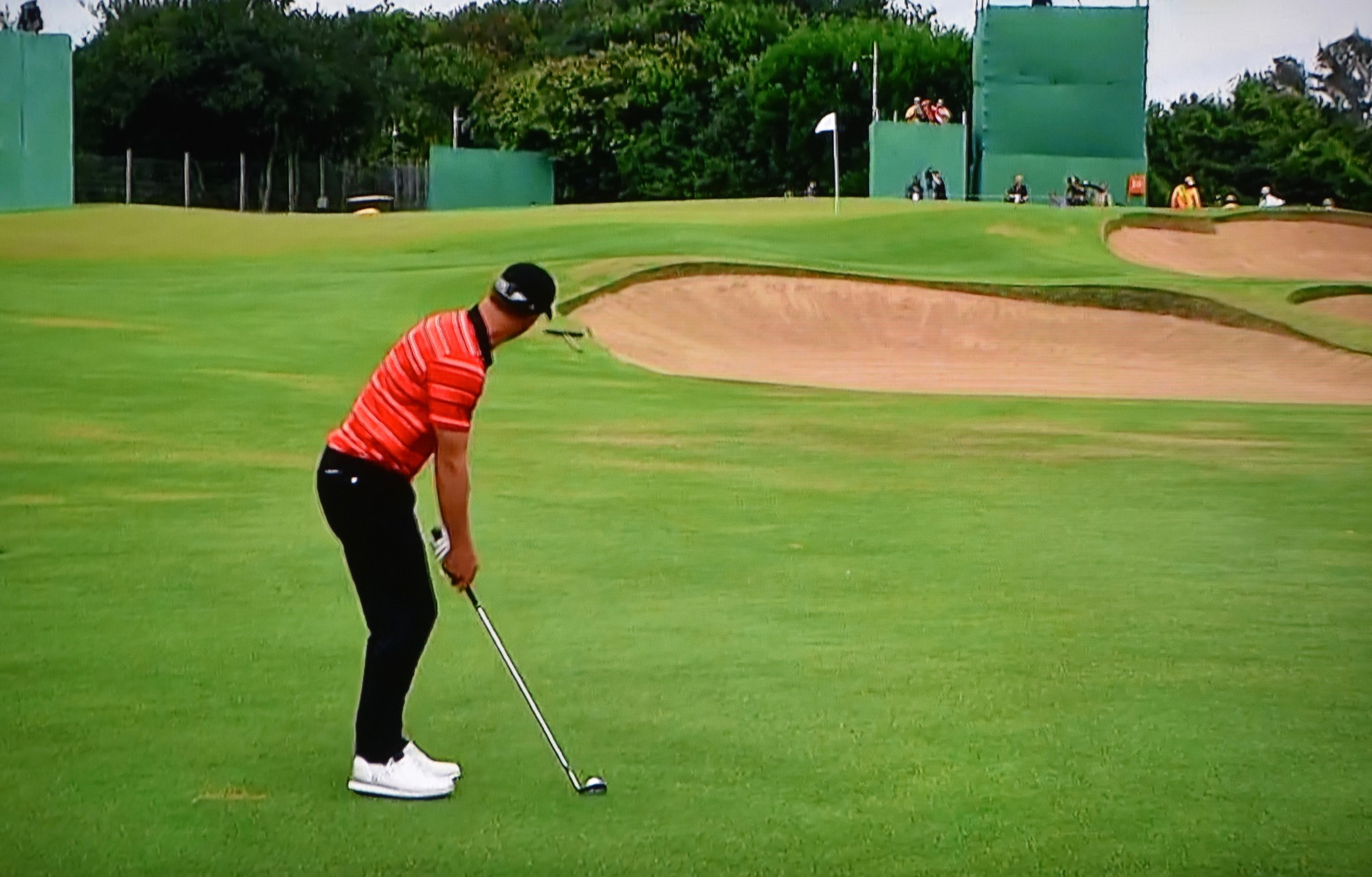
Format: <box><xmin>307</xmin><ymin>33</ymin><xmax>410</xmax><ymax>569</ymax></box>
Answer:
<box><xmin>815</xmin><ymin>112</ymin><xmax>834</xmax><ymax>133</ymax></box>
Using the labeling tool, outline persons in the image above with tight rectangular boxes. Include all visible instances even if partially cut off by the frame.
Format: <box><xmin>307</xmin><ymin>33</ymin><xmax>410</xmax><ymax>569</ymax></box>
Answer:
<box><xmin>921</xmin><ymin>99</ymin><xmax>940</xmax><ymax>124</ymax></box>
<box><xmin>930</xmin><ymin>170</ymin><xmax>947</xmax><ymax>200</ymax></box>
<box><xmin>1258</xmin><ymin>187</ymin><xmax>1286</xmax><ymax>207</ymax></box>
<box><xmin>1222</xmin><ymin>194</ymin><xmax>1241</xmax><ymax>209</ymax></box>
<box><xmin>1006</xmin><ymin>175</ymin><xmax>1028</xmax><ymax>203</ymax></box>
<box><xmin>934</xmin><ymin>98</ymin><xmax>953</xmax><ymax>123</ymax></box>
<box><xmin>803</xmin><ymin>181</ymin><xmax>818</xmax><ymax>196</ymax></box>
<box><xmin>1171</xmin><ymin>175</ymin><xmax>1202</xmax><ymax>210</ymax></box>
<box><xmin>1049</xmin><ymin>176</ymin><xmax>1113</xmax><ymax>207</ymax></box>
<box><xmin>907</xmin><ymin>175</ymin><xmax>924</xmax><ymax>200</ymax></box>
<box><xmin>905</xmin><ymin>97</ymin><xmax>929</xmax><ymax>123</ymax></box>
<box><xmin>315</xmin><ymin>261</ymin><xmax>555</xmax><ymax>797</ymax></box>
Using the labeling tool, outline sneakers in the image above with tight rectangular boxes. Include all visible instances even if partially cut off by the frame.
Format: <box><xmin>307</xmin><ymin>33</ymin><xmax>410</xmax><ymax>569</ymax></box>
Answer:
<box><xmin>400</xmin><ymin>740</ymin><xmax>461</xmax><ymax>780</ymax></box>
<box><xmin>348</xmin><ymin>751</ymin><xmax>455</xmax><ymax>798</ymax></box>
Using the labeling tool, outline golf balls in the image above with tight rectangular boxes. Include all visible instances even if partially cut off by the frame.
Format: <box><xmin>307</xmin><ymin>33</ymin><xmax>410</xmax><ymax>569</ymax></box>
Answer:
<box><xmin>584</xmin><ymin>776</ymin><xmax>607</xmax><ymax>797</ymax></box>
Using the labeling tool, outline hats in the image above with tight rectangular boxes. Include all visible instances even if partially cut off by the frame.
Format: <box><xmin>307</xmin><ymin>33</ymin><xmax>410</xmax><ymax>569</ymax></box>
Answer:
<box><xmin>492</xmin><ymin>263</ymin><xmax>555</xmax><ymax>321</ymax></box>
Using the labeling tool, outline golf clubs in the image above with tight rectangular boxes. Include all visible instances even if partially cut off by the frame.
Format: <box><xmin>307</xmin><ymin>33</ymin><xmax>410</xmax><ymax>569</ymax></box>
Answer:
<box><xmin>431</xmin><ymin>524</ymin><xmax>609</xmax><ymax>796</ymax></box>
